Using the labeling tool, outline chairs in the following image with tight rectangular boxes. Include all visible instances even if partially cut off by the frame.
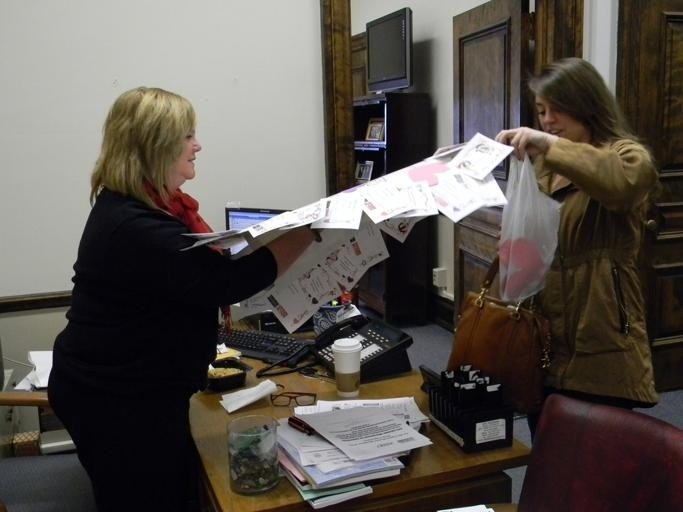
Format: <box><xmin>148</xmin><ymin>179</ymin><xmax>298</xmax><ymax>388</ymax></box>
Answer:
<box><xmin>1</xmin><ymin>338</ymin><xmax>96</xmax><ymax>512</ymax></box>
<box><xmin>517</xmin><ymin>394</ymin><xmax>683</xmax><ymax>511</ymax></box>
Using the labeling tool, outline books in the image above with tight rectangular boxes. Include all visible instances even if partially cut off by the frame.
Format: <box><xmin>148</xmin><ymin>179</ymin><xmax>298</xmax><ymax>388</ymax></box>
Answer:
<box><xmin>267</xmin><ymin>396</ymin><xmax>433</xmax><ymax>511</ymax></box>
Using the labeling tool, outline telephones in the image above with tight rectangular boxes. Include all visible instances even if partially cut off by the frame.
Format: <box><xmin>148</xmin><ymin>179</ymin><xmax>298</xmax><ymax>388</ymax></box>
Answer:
<box><xmin>309</xmin><ymin>315</ymin><xmax>413</xmax><ymax>383</ymax></box>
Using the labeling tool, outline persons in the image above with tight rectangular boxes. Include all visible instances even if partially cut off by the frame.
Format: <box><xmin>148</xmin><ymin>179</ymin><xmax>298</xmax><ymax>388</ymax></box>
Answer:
<box><xmin>40</xmin><ymin>83</ymin><xmax>323</xmax><ymax>511</ymax></box>
<box><xmin>492</xmin><ymin>50</ymin><xmax>663</xmax><ymax>451</ymax></box>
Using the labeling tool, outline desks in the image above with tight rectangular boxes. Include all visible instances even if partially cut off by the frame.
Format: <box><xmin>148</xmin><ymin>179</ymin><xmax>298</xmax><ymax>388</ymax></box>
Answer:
<box><xmin>189</xmin><ymin>319</ymin><xmax>530</xmax><ymax>512</ymax></box>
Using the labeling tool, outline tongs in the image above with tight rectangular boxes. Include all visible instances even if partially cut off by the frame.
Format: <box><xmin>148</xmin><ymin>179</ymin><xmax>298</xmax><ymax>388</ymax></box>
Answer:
<box><xmin>255</xmin><ymin>343</ymin><xmax>318</xmax><ymax>376</ymax></box>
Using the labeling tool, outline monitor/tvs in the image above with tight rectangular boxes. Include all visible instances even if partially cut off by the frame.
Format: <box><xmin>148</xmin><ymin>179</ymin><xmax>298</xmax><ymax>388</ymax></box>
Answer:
<box><xmin>366</xmin><ymin>8</ymin><xmax>413</xmax><ymax>91</ymax></box>
<box><xmin>225</xmin><ymin>206</ymin><xmax>292</xmax><ymax>232</ymax></box>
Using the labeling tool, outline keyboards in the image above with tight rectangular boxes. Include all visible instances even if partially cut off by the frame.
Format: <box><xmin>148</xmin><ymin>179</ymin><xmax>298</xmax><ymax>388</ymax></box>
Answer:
<box><xmin>217</xmin><ymin>326</ymin><xmax>311</xmax><ymax>367</ymax></box>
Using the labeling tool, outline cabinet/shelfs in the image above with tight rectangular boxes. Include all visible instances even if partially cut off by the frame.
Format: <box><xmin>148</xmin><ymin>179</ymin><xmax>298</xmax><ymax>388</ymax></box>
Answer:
<box><xmin>351</xmin><ymin>91</ymin><xmax>439</xmax><ymax>320</ymax></box>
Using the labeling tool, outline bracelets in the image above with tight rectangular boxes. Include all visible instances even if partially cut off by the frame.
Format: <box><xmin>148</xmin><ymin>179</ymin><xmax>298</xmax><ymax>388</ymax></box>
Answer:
<box><xmin>305</xmin><ymin>221</ymin><xmax>322</xmax><ymax>244</ymax></box>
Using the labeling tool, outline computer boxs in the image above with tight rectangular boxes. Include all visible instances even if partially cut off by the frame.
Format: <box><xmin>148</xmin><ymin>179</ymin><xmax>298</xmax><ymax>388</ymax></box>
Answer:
<box><xmin>246</xmin><ymin>307</ymin><xmax>313</xmax><ymax>334</ymax></box>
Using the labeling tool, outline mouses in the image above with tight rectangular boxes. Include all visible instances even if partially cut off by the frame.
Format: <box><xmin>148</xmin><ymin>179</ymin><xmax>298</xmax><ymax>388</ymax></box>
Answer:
<box><xmin>217</xmin><ymin>355</ymin><xmax>250</xmax><ymax>371</ymax></box>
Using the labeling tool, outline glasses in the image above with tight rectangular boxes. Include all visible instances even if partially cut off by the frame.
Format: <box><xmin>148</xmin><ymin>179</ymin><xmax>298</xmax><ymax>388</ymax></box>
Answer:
<box><xmin>271</xmin><ymin>382</ymin><xmax>316</xmax><ymax>407</ymax></box>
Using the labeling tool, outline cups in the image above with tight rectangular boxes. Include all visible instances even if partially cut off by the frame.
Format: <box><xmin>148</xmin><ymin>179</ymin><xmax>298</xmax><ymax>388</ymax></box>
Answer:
<box><xmin>331</xmin><ymin>337</ymin><xmax>361</xmax><ymax>398</ymax></box>
<box><xmin>227</xmin><ymin>415</ymin><xmax>279</xmax><ymax>494</ymax></box>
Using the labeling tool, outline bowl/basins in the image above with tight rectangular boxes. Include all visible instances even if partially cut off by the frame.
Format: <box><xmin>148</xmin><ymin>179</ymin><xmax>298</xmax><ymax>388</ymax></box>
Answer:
<box><xmin>203</xmin><ymin>355</ymin><xmax>251</xmax><ymax>392</ymax></box>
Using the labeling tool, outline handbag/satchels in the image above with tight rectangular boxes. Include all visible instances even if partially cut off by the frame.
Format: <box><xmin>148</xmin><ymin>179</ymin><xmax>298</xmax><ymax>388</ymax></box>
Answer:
<box><xmin>446</xmin><ymin>258</ymin><xmax>550</xmax><ymax>416</ymax></box>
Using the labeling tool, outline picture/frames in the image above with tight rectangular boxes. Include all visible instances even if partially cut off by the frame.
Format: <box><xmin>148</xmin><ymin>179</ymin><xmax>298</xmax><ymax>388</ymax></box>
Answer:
<box><xmin>364</xmin><ymin>118</ymin><xmax>389</xmax><ymax>141</ymax></box>
<box><xmin>356</xmin><ymin>158</ymin><xmax>376</xmax><ymax>183</ymax></box>
